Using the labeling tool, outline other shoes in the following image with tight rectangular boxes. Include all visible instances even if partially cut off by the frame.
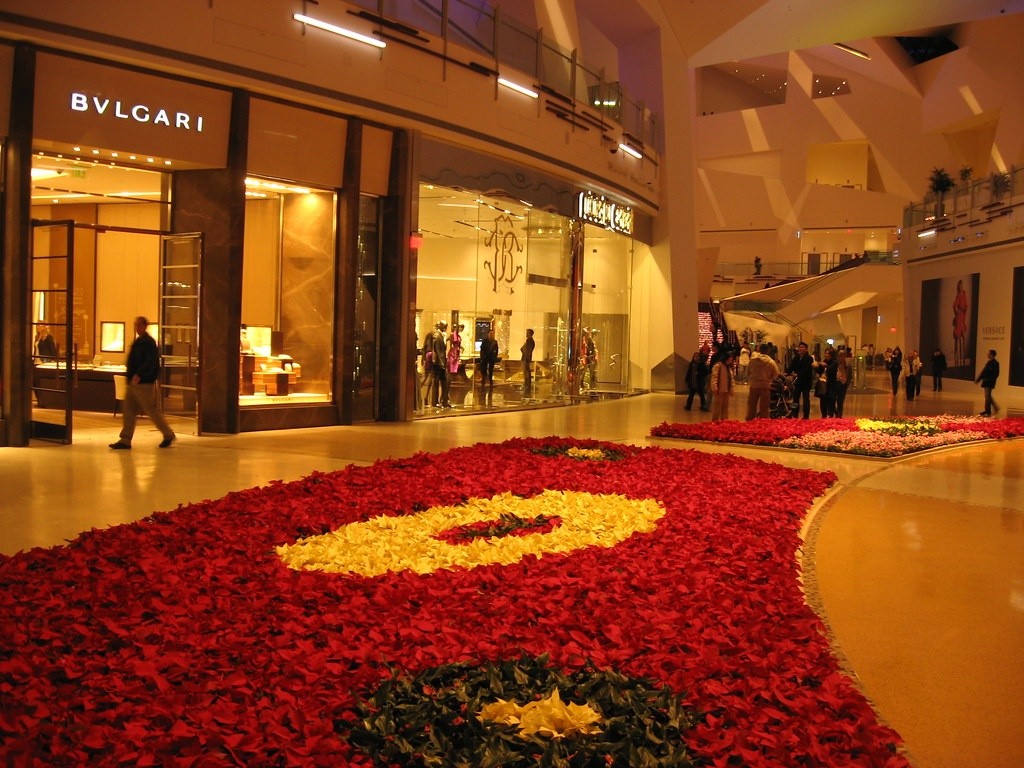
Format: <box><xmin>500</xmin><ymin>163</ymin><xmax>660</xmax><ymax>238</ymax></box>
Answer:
<box><xmin>108</xmin><ymin>441</ymin><xmax>131</xmax><ymax>449</ymax></box>
<box><xmin>979</xmin><ymin>410</ymin><xmax>990</xmax><ymax>415</ymax></box>
<box><xmin>700</xmin><ymin>406</ymin><xmax>709</xmax><ymax>412</ymax></box>
<box><xmin>433</xmin><ymin>403</ymin><xmax>441</xmax><ymax>407</ymax></box>
<box><xmin>158</xmin><ymin>434</ymin><xmax>176</xmax><ymax>448</ymax></box>
<box><xmin>791</xmin><ymin>412</ymin><xmax>798</xmax><ymax>418</ymax></box>
<box><xmin>441</xmin><ymin>403</ymin><xmax>454</xmax><ymax>408</ymax></box>
<box><xmin>684</xmin><ymin>406</ymin><xmax>692</xmax><ymax>411</ymax></box>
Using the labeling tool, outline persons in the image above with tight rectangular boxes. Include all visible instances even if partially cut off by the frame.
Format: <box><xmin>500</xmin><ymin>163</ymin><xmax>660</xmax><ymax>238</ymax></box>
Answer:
<box><xmin>951</xmin><ymin>280</ymin><xmax>967</xmax><ymax>368</ymax></box>
<box><xmin>109</xmin><ymin>317</ymin><xmax>175</xmax><ymax>448</ymax></box>
<box><xmin>419</xmin><ymin>321</ymin><xmax>464</xmax><ymax>409</ymax></box>
<box><xmin>579</xmin><ymin>328</ymin><xmax>588</xmax><ymax>390</ymax></box>
<box><xmin>684</xmin><ymin>341</ymin><xmax>922</xmax><ymax>421</ymax></box>
<box><xmin>930</xmin><ymin>349</ymin><xmax>946</xmax><ymax>392</ymax></box>
<box><xmin>521</xmin><ymin>329</ymin><xmax>535</xmax><ymax>395</ymax></box>
<box><xmin>753</xmin><ymin>257</ymin><xmax>762</xmax><ymax>276</ymax></box>
<box><xmin>587</xmin><ymin>329</ymin><xmax>598</xmax><ymax>391</ymax></box>
<box><xmin>34</xmin><ymin>320</ymin><xmax>57</xmax><ymax>363</ymax></box>
<box><xmin>480</xmin><ymin>330</ymin><xmax>499</xmax><ymax>385</ymax></box>
<box><xmin>975</xmin><ymin>350</ymin><xmax>999</xmax><ymax>416</ymax></box>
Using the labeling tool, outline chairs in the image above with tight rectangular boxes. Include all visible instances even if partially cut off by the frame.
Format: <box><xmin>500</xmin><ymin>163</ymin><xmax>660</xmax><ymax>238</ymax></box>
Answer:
<box><xmin>113</xmin><ymin>375</ymin><xmax>132</xmax><ymax>417</ymax></box>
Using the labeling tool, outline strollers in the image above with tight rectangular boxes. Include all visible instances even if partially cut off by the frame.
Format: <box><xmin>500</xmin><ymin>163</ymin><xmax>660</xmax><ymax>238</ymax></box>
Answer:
<box><xmin>756</xmin><ymin>373</ymin><xmax>799</xmax><ymax>418</ymax></box>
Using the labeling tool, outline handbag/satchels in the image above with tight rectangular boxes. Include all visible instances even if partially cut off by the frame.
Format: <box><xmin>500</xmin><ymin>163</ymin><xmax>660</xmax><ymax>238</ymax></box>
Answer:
<box><xmin>814</xmin><ymin>377</ymin><xmax>826</xmax><ymax>396</ymax></box>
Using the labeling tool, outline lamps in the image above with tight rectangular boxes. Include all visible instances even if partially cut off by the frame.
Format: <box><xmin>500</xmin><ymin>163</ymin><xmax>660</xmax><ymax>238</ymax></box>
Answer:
<box><xmin>618</xmin><ymin>141</ymin><xmax>644</xmax><ymax>160</ymax></box>
<box><xmin>496</xmin><ymin>78</ymin><xmax>538</xmax><ymax>102</ymax></box>
<box><xmin>293</xmin><ymin>12</ymin><xmax>387</xmax><ymax>51</ymax></box>
<box><xmin>833</xmin><ymin>43</ymin><xmax>872</xmax><ymax>61</ymax></box>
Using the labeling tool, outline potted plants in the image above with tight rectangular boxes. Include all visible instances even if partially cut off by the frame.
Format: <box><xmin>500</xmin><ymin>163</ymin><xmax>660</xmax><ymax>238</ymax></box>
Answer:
<box><xmin>960</xmin><ymin>166</ymin><xmax>973</xmax><ymax>193</ymax></box>
<box><xmin>989</xmin><ymin>173</ymin><xmax>1012</xmax><ymax>203</ymax></box>
<box><xmin>929</xmin><ymin>168</ymin><xmax>953</xmax><ymax>220</ymax></box>
